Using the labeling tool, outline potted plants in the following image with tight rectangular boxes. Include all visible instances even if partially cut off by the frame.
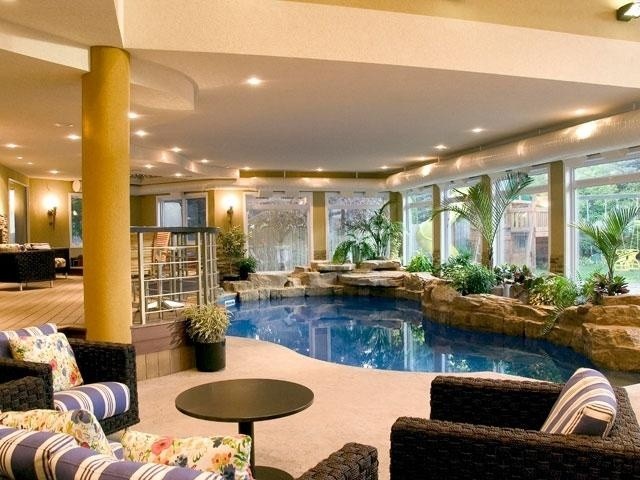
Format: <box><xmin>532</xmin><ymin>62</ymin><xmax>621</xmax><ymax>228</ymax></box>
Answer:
<box><xmin>426</xmin><ymin>170</ymin><xmax>639</xmax><ymax>308</ymax></box>
<box><xmin>214</xmin><ymin>205</ymin><xmax>256</xmax><ymax>285</ymax></box>
<box><xmin>178</xmin><ymin>301</ymin><xmax>234</xmax><ymax>373</ymax></box>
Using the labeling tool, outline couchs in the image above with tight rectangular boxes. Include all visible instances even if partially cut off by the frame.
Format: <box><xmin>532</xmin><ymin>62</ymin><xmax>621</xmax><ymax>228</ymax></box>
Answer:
<box><xmin>0</xmin><ymin>243</ymin><xmax>56</xmax><ymax>291</ymax></box>
<box><xmin>0</xmin><ymin>377</ymin><xmax>379</xmax><ymax>479</ymax></box>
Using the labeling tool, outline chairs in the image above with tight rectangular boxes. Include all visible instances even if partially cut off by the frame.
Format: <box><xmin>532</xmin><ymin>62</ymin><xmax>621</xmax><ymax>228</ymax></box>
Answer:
<box><xmin>0</xmin><ymin>322</ymin><xmax>141</xmax><ymax>436</ymax></box>
<box><xmin>125</xmin><ymin>230</ymin><xmax>174</xmax><ymax>323</ymax></box>
<box><xmin>51</xmin><ymin>248</ymin><xmax>70</xmax><ymax>279</ymax></box>
<box><xmin>388</xmin><ymin>366</ymin><xmax>639</xmax><ymax>480</ymax></box>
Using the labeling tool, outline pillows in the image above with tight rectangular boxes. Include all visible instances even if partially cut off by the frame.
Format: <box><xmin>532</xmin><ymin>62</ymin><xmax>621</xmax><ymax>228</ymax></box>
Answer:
<box><xmin>0</xmin><ymin>408</ymin><xmax>257</xmax><ymax>480</ymax></box>
<box><xmin>8</xmin><ymin>332</ymin><xmax>84</xmax><ymax>393</ymax></box>
